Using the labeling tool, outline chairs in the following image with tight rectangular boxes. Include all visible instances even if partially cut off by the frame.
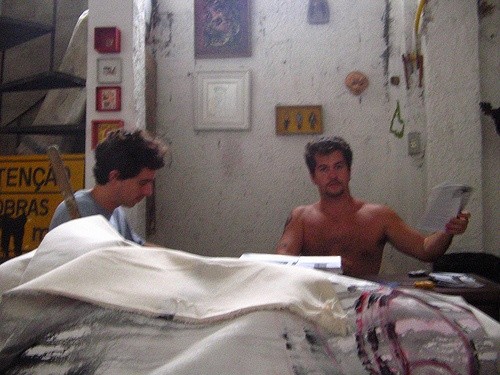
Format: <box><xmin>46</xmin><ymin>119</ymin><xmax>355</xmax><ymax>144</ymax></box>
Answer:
<box><xmin>431</xmin><ymin>252</ymin><xmax>500</xmax><ymax>284</ymax></box>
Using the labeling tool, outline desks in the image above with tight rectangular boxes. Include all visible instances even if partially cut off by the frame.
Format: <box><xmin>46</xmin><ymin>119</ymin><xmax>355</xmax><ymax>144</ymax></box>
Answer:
<box><xmin>359</xmin><ymin>273</ymin><xmax>499</xmax><ymax>300</ymax></box>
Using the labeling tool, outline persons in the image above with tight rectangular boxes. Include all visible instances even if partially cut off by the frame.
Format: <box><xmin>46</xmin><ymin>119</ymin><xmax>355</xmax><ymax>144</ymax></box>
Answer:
<box><xmin>48</xmin><ymin>126</ymin><xmax>166</xmax><ymax>250</ymax></box>
<box><xmin>275</xmin><ymin>135</ymin><xmax>471</xmax><ymax>276</ymax></box>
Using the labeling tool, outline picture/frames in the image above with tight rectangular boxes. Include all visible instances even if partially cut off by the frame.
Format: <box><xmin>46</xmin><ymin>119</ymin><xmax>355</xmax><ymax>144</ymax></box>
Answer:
<box><xmin>190</xmin><ymin>69</ymin><xmax>252</xmax><ymax>131</ymax></box>
<box><xmin>96</xmin><ymin>86</ymin><xmax>122</xmax><ymax>112</ymax></box>
<box><xmin>276</xmin><ymin>105</ymin><xmax>324</xmax><ymax>135</ymax></box>
<box><xmin>193</xmin><ymin>0</ymin><xmax>251</xmax><ymax>60</ymax></box>
<box><xmin>96</xmin><ymin>57</ymin><xmax>122</xmax><ymax>84</ymax></box>
<box><xmin>94</xmin><ymin>26</ymin><xmax>120</xmax><ymax>53</ymax></box>
<box><xmin>91</xmin><ymin>119</ymin><xmax>124</xmax><ymax>151</ymax></box>
